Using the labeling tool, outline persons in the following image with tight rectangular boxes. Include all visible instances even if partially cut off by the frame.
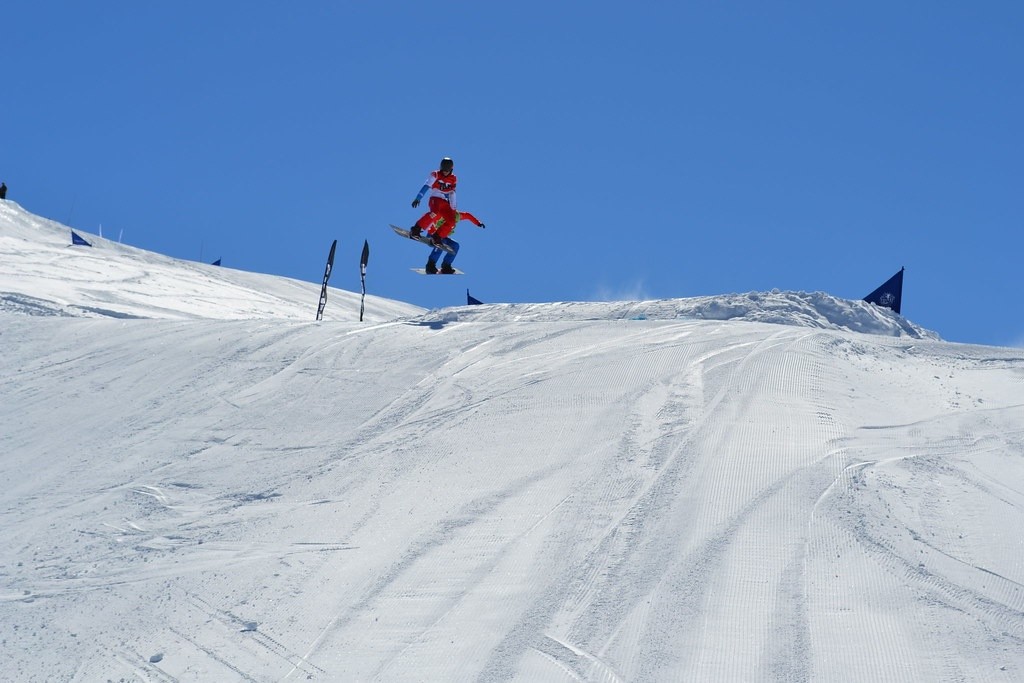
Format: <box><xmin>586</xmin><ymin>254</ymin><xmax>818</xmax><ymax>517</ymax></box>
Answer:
<box><xmin>426</xmin><ymin>204</ymin><xmax>485</xmax><ymax>275</ymax></box>
<box><xmin>410</xmin><ymin>158</ymin><xmax>456</xmax><ymax>248</ymax></box>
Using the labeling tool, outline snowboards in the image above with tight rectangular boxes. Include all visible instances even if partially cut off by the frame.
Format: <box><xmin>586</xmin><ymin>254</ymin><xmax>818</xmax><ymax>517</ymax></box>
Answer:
<box><xmin>388</xmin><ymin>223</ymin><xmax>456</xmax><ymax>255</ymax></box>
<box><xmin>409</xmin><ymin>266</ymin><xmax>465</xmax><ymax>275</ymax></box>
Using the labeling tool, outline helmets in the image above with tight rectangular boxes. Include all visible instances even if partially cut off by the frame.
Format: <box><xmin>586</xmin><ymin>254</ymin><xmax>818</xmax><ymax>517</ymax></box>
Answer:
<box><xmin>439</xmin><ymin>158</ymin><xmax>453</xmax><ymax>172</ymax></box>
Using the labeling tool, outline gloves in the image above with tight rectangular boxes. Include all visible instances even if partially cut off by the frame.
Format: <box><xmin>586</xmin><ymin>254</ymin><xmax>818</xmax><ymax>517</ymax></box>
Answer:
<box><xmin>478</xmin><ymin>223</ymin><xmax>485</xmax><ymax>229</ymax></box>
<box><xmin>412</xmin><ymin>199</ymin><xmax>420</xmax><ymax>208</ymax></box>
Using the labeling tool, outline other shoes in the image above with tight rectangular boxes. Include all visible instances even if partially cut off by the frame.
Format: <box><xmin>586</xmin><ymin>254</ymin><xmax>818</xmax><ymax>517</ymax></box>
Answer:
<box><xmin>409</xmin><ymin>226</ymin><xmax>421</xmax><ymax>240</ymax></box>
<box><xmin>440</xmin><ymin>262</ymin><xmax>455</xmax><ymax>273</ymax></box>
<box><xmin>425</xmin><ymin>259</ymin><xmax>438</xmax><ymax>274</ymax></box>
<box><xmin>432</xmin><ymin>233</ymin><xmax>442</xmax><ymax>249</ymax></box>
